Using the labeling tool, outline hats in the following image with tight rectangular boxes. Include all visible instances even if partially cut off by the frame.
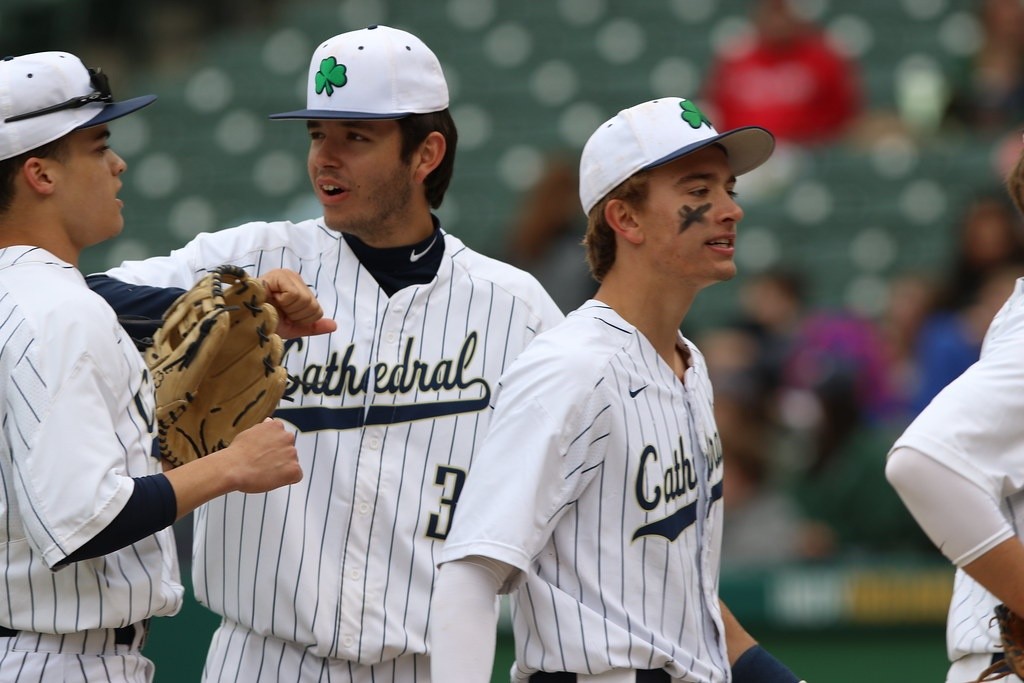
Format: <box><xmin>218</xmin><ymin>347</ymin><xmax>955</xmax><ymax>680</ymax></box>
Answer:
<box><xmin>0</xmin><ymin>51</ymin><xmax>160</xmax><ymax>161</ymax></box>
<box><xmin>268</xmin><ymin>23</ymin><xmax>450</xmax><ymax>119</ymax></box>
<box><xmin>579</xmin><ymin>97</ymin><xmax>776</xmax><ymax>218</ymax></box>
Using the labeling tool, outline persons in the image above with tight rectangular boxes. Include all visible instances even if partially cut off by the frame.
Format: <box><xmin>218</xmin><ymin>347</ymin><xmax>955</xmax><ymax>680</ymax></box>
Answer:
<box><xmin>83</xmin><ymin>25</ymin><xmax>568</xmax><ymax>683</ymax></box>
<box><xmin>699</xmin><ymin>0</ymin><xmax>1024</xmax><ymax>516</ymax></box>
<box><xmin>886</xmin><ymin>153</ymin><xmax>1024</xmax><ymax>683</ymax></box>
<box><xmin>0</xmin><ymin>50</ymin><xmax>302</xmax><ymax>683</ymax></box>
<box><xmin>705</xmin><ymin>0</ymin><xmax>863</xmax><ymax>142</ymax></box>
<box><xmin>427</xmin><ymin>94</ymin><xmax>805</xmax><ymax>683</ymax></box>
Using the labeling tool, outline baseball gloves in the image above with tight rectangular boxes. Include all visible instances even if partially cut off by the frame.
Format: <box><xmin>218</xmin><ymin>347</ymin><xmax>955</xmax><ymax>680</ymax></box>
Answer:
<box><xmin>138</xmin><ymin>263</ymin><xmax>295</xmax><ymax>462</ymax></box>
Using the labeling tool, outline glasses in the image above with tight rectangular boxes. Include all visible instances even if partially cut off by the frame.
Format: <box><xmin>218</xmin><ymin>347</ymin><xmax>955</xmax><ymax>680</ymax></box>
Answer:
<box><xmin>4</xmin><ymin>71</ymin><xmax>114</xmax><ymax>125</ymax></box>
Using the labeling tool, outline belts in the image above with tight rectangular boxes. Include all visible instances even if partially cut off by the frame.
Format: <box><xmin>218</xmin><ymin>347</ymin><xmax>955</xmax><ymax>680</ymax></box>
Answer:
<box><xmin>0</xmin><ymin>624</ymin><xmax>137</xmax><ymax>645</ymax></box>
<box><xmin>990</xmin><ymin>651</ymin><xmax>1012</xmax><ymax>673</ymax></box>
<box><xmin>528</xmin><ymin>668</ymin><xmax>672</xmax><ymax>683</ymax></box>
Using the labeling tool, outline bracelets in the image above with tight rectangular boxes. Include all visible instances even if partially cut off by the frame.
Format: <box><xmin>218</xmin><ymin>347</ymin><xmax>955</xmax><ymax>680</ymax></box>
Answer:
<box><xmin>732</xmin><ymin>644</ymin><xmax>801</xmax><ymax>682</ymax></box>
